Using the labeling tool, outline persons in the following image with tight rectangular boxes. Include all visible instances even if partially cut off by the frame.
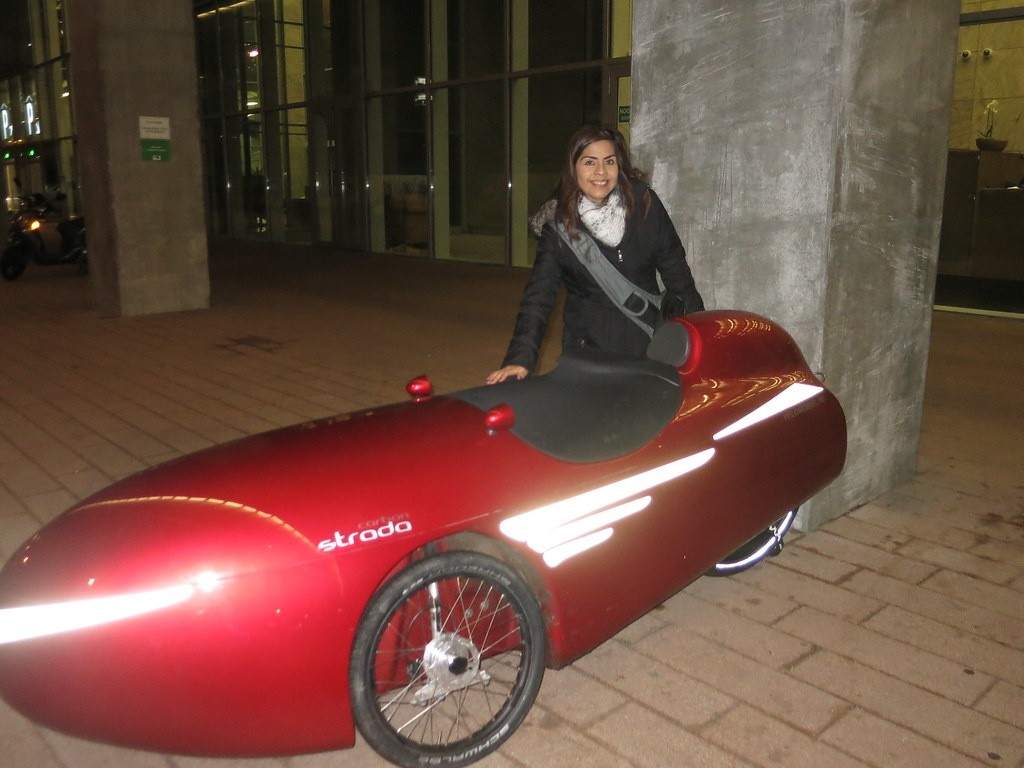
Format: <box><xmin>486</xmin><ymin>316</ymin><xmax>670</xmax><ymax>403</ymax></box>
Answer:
<box><xmin>486</xmin><ymin>125</ymin><xmax>705</xmax><ymax>386</ymax></box>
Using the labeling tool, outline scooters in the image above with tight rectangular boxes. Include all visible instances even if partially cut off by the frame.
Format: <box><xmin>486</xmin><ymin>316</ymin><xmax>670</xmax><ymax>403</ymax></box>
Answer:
<box><xmin>0</xmin><ymin>174</ymin><xmax>89</xmax><ymax>282</ymax></box>
